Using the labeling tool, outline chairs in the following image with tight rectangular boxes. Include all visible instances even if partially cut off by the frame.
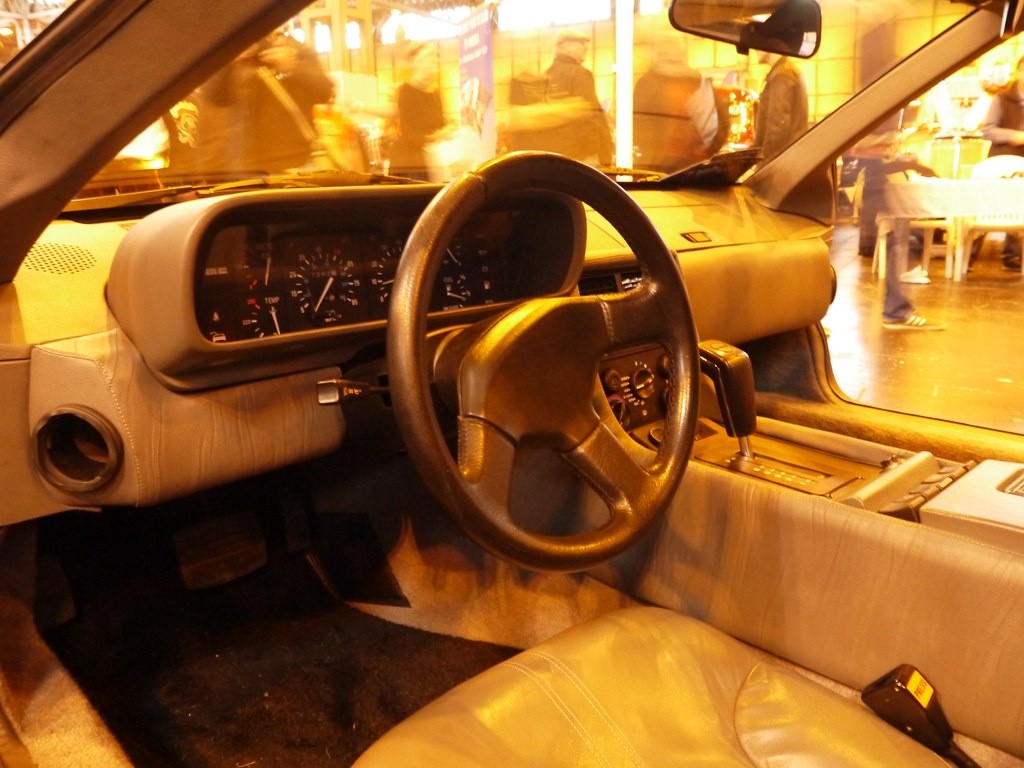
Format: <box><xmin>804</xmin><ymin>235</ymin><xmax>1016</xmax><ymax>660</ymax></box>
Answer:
<box><xmin>345</xmin><ymin>604</ymin><xmax>968</xmax><ymax>768</ymax></box>
<box><xmin>869</xmin><ymin>165</ymin><xmax>956</xmax><ymax>281</ymax></box>
<box><xmin>950</xmin><ymin>154</ymin><xmax>1024</xmax><ymax>283</ymax></box>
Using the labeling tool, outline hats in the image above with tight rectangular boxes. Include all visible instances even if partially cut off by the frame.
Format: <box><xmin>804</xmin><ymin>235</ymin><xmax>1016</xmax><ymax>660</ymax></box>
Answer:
<box><xmin>555</xmin><ymin>29</ymin><xmax>590</xmax><ymax>45</ymax></box>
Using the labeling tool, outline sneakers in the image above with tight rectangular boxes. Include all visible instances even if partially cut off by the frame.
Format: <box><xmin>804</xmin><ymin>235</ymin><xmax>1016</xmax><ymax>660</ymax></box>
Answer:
<box><xmin>882</xmin><ymin>312</ymin><xmax>945</xmax><ymax>330</ymax></box>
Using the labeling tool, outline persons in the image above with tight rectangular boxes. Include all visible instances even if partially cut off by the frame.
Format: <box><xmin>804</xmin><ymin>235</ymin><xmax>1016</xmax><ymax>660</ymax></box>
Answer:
<box><xmin>391</xmin><ymin>38</ymin><xmax>456</xmax><ymax>174</ymax></box>
<box><xmin>968</xmin><ymin>56</ymin><xmax>1024</xmax><ymax>274</ymax></box>
<box><xmin>853</xmin><ymin>96</ymin><xmax>946</xmax><ymax>332</ymax></box>
<box><xmin>199</xmin><ymin>28</ymin><xmax>336</xmax><ymax>170</ymax></box>
<box><xmin>632</xmin><ymin>33</ymin><xmax>721</xmax><ymax>174</ymax></box>
<box><xmin>539</xmin><ymin>27</ymin><xmax>616</xmax><ymax>165</ymax></box>
<box><xmin>748</xmin><ymin>44</ymin><xmax>811</xmax><ymax>175</ymax></box>
<box><xmin>311</xmin><ymin>79</ymin><xmax>372</xmax><ymax>175</ymax></box>
